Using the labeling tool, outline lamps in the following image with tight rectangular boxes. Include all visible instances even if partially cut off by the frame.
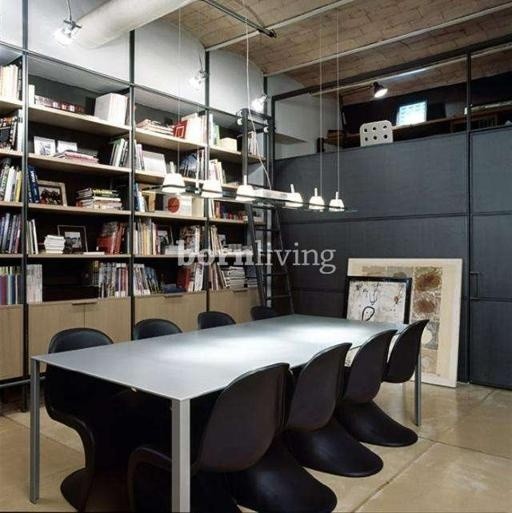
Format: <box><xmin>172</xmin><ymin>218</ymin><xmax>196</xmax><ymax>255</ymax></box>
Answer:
<box><xmin>329</xmin><ymin>7</ymin><xmax>344</xmax><ymax>211</ymax></box>
<box><xmin>162</xmin><ymin>8</ymin><xmax>186</xmax><ymax>194</ymax></box>
<box><xmin>340</xmin><ymin>81</ymin><xmax>387</xmax><ymax>136</ymax></box>
<box><xmin>54</xmin><ymin>0</ymin><xmax>82</xmax><ymax>46</ymax></box>
<box><xmin>188</xmin><ymin>45</ymin><xmax>209</xmax><ymax>91</ymax></box>
<box><xmin>284</xmin><ymin>184</ymin><xmax>303</xmax><ymax>209</ymax></box>
<box><xmin>308</xmin><ymin>13</ymin><xmax>324</xmax><ymax>210</ymax></box>
<box><xmin>200</xmin><ymin>165</ymin><xmax>223</xmax><ymax>199</ymax></box>
<box><xmin>235</xmin><ymin>176</ymin><xmax>255</xmax><ymax>201</ymax></box>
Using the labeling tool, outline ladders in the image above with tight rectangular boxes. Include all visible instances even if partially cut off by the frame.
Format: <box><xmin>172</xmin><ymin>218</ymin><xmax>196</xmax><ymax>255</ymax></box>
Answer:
<box><xmin>245</xmin><ymin>204</ymin><xmax>295</xmax><ymax>313</ymax></box>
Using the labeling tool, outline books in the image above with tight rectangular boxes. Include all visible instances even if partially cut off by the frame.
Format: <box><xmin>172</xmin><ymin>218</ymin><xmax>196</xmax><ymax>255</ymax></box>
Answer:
<box><xmin>97</xmin><ymin>221</ymin><xmax>127</xmax><ymax>253</ymax></box>
<box><xmin>176</xmin><ymin>264</ymin><xmax>258</xmax><ymax>292</ymax></box>
<box><xmin>28</xmin><ymin>84</ymin><xmax>35</xmax><ymax>104</ymax></box>
<box><xmin>0</xmin><ymin>107</ymin><xmax>25</xmax><ymax>151</ymax></box>
<box><xmin>207</xmin><ymin>197</ymin><xmax>245</xmax><ymax>221</ymax></box>
<box><xmin>52</xmin><ymin>149</ymin><xmax>97</xmax><ymax>164</ymax></box>
<box><xmin>0</xmin><ymin>212</ymin><xmax>22</xmax><ymax>253</ymax></box>
<box><xmin>179</xmin><ymin>149</ymin><xmax>204</xmax><ymax>179</ymax></box>
<box><xmin>209</xmin><ymin>113</ymin><xmax>238</xmax><ymax>151</ymax></box>
<box><xmin>57</xmin><ymin>140</ymin><xmax>78</xmax><ymax>152</ymax></box>
<box><xmin>133</xmin><ymin>140</ymin><xmax>166</xmax><ymax>173</ymax></box>
<box><xmin>1</xmin><ymin>158</ymin><xmax>22</xmax><ymax>203</ymax></box>
<box><xmin>27</xmin><ymin>219</ymin><xmax>39</xmax><ymax>254</ymax></box>
<box><xmin>99</xmin><ymin>137</ymin><xmax>129</xmax><ymax>168</ymax></box>
<box><xmin>80</xmin><ymin>260</ymin><xmax>128</xmax><ymax>298</ymax></box>
<box><xmin>180</xmin><ymin>224</ymin><xmax>224</xmax><ymax>253</ymax></box>
<box><xmin>181</xmin><ymin>113</ymin><xmax>207</xmax><ymax>142</ymax></box>
<box><xmin>1</xmin><ymin>64</ymin><xmax>21</xmax><ymax>100</ymax></box>
<box><xmin>134</xmin><ymin>182</ymin><xmax>149</xmax><ymax>211</ymax></box>
<box><xmin>209</xmin><ymin>158</ymin><xmax>227</xmax><ymax>183</ymax></box>
<box><xmin>133</xmin><ymin>217</ymin><xmax>158</xmax><ymax>255</ymax></box>
<box><xmin>75</xmin><ymin>187</ymin><xmax>122</xmax><ymax>210</ymax></box>
<box><xmin>27</xmin><ymin>164</ymin><xmax>42</xmax><ymax>203</ymax></box>
<box><xmin>248</xmin><ymin>130</ymin><xmax>263</xmax><ymax>157</ymax></box>
<box><xmin>136</xmin><ymin>118</ymin><xmax>175</xmax><ymax>135</ymax></box>
<box><xmin>164</xmin><ymin>193</ymin><xmax>192</xmax><ymax>215</ymax></box>
<box><xmin>0</xmin><ymin>264</ymin><xmax>42</xmax><ymax>305</ymax></box>
<box><xmin>44</xmin><ymin>235</ymin><xmax>65</xmax><ymax>254</ymax></box>
<box><xmin>133</xmin><ymin>264</ymin><xmax>161</xmax><ymax>296</ymax></box>
<box><xmin>95</xmin><ymin>93</ymin><xmax>127</xmax><ymax>125</ymax></box>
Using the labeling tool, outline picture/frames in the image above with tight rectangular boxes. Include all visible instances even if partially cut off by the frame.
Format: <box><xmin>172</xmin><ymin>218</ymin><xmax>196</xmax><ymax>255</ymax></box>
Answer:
<box><xmin>37</xmin><ymin>180</ymin><xmax>68</xmax><ymax>208</ymax></box>
<box><xmin>347</xmin><ymin>257</ymin><xmax>462</xmax><ymax>388</ymax></box>
<box><xmin>57</xmin><ymin>138</ymin><xmax>78</xmax><ymax>153</ymax></box>
<box><xmin>57</xmin><ymin>224</ymin><xmax>88</xmax><ymax>256</ymax></box>
<box><xmin>154</xmin><ymin>223</ymin><xmax>174</xmax><ymax>249</ymax></box>
<box><xmin>341</xmin><ymin>275</ymin><xmax>413</xmax><ymax>372</ymax></box>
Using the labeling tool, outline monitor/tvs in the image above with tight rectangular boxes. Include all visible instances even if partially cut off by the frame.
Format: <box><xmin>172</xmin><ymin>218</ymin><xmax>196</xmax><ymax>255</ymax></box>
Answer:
<box><xmin>399</xmin><ymin>99</ymin><xmax>427</xmax><ymax>124</ymax></box>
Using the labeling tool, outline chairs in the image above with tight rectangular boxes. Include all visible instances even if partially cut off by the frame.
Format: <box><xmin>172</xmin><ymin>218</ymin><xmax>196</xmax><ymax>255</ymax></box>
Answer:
<box><xmin>250</xmin><ymin>306</ymin><xmax>276</xmax><ymax>321</ymax></box>
<box><xmin>43</xmin><ymin>328</ymin><xmax>164</xmax><ymax>513</ymax></box>
<box><xmin>131</xmin><ymin>318</ymin><xmax>218</xmax><ymax>445</ymax></box>
<box><xmin>334</xmin><ymin>319</ymin><xmax>429</xmax><ymax>447</ymax></box>
<box><xmin>285</xmin><ymin>329</ymin><xmax>397</xmax><ymax>478</ymax></box>
<box><xmin>224</xmin><ymin>342</ymin><xmax>352</xmax><ymax>513</ymax></box>
<box><xmin>126</xmin><ymin>363</ymin><xmax>289</xmax><ymax>513</ymax></box>
<box><xmin>197</xmin><ymin>311</ymin><xmax>235</xmax><ymax>332</ymax></box>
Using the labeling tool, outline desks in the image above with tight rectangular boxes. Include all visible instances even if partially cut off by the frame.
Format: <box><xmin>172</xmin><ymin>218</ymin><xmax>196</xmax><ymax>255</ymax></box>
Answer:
<box><xmin>29</xmin><ymin>314</ymin><xmax>421</xmax><ymax>513</ymax></box>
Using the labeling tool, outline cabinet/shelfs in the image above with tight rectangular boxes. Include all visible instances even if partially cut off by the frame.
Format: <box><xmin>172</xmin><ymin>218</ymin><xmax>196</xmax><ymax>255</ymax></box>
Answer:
<box><xmin>28</xmin><ymin>297</ymin><xmax>132</xmax><ymax>375</ymax></box>
<box><xmin>0</xmin><ymin>39</ymin><xmax>26</xmax><ymax>308</ymax></box>
<box><xmin>321</xmin><ymin>103</ymin><xmax>512</xmax><ymax>152</ymax></box>
<box><xmin>208</xmin><ymin>290</ymin><xmax>264</xmax><ymax>329</ymax></box>
<box><xmin>208</xmin><ymin>107</ymin><xmax>268</xmax><ymax>291</ymax></box>
<box><xmin>130</xmin><ymin>82</ymin><xmax>211</xmax><ymax>297</ymax></box>
<box><xmin>25</xmin><ymin>48</ymin><xmax>134</xmax><ymax>304</ymax></box>
<box><xmin>0</xmin><ymin>303</ymin><xmax>23</xmax><ymax>382</ymax></box>
<box><xmin>133</xmin><ymin>291</ymin><xmax>206</xmax><ymax>333</ymax></box>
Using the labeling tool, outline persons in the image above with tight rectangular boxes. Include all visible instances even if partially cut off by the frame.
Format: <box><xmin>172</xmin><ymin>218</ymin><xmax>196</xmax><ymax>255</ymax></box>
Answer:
<box><xmin>40</xmin><ymin>189</ymin><xmax>63</xmax><ymax>203</ymax></box>
<box><xmin>67</xmin><ymin>236</ymin><xmax>81</xmax><ymax>247</ymax></box>
